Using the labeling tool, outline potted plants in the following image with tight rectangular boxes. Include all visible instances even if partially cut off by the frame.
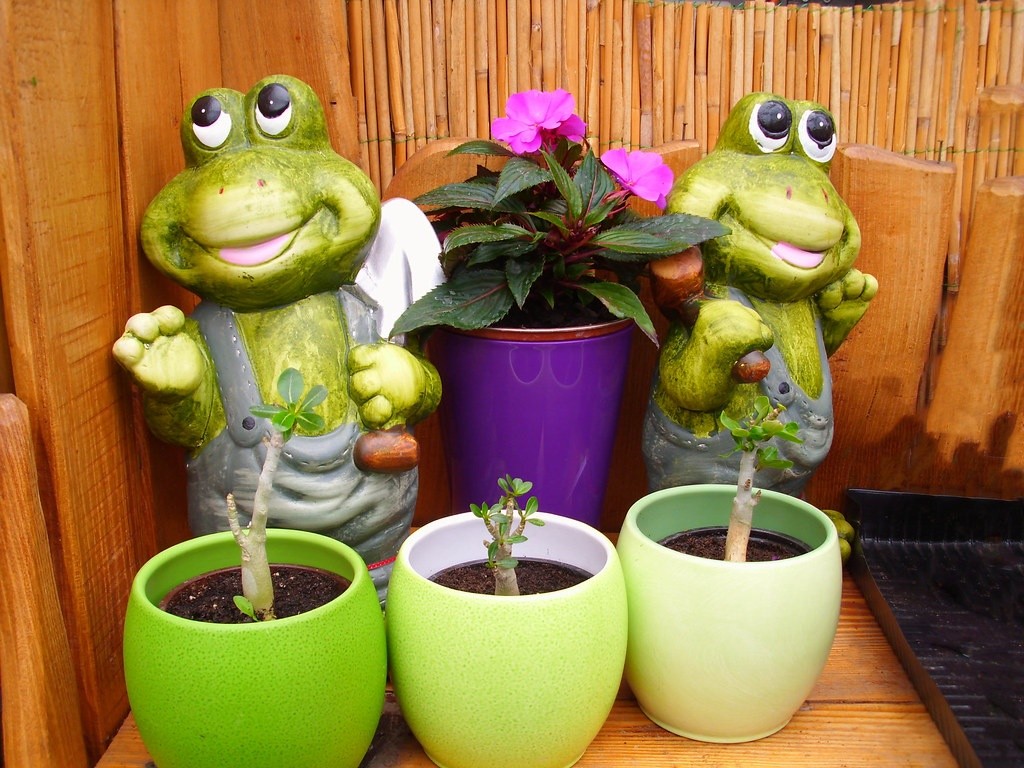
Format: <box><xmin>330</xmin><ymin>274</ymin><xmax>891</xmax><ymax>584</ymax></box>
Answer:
<box><xmin>616</xmin><ymin>392</ymin><xmax>842</xmax><ymax>745</ymax></box>
<box><xmin>386</xmin><ymin>474</ymin><xmax>628</xmax><ymax>768</ymax></box>
<box><xmin>123</xmin><ymin>368</ymin><xmax>388</xmax><ymax>768</ymax></box>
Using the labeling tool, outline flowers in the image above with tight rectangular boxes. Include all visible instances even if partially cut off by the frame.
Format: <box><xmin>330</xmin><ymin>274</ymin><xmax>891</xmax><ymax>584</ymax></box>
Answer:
<box><xmin>385</xmin><ymin>90</ymin><xmax>732</xmax><ymax>351</ymax></box>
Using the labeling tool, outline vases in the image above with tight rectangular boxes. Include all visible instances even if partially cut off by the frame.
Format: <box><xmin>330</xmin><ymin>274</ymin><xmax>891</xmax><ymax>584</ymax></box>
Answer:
<box><xmin>437</xmin><ymin>321</ymin><xmax>632</xmax><ymax>529</ymax></box>
